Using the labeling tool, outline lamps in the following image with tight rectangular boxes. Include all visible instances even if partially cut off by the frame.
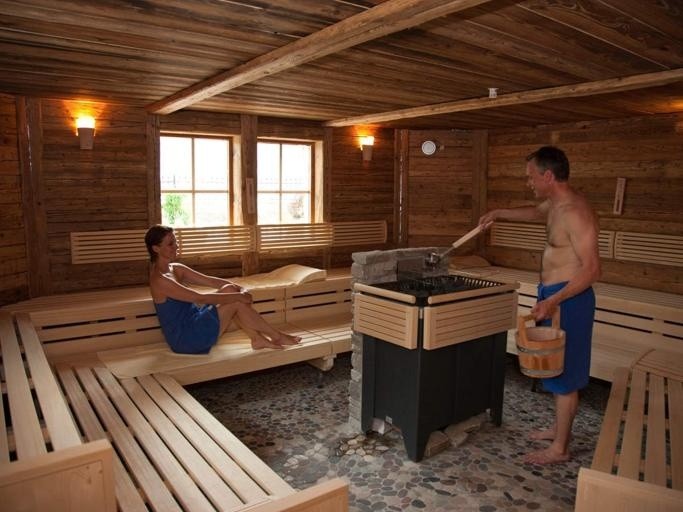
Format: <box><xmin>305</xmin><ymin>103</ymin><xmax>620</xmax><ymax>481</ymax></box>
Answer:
<box><xmin>79</xmin><ymin>127</ymin><xmax>94</xmax><ymax>149</ymax></box>
<box><xmin>362</xmin><ymin>144</ymin><xmax>373</xmax><ymax>161</ymax></box>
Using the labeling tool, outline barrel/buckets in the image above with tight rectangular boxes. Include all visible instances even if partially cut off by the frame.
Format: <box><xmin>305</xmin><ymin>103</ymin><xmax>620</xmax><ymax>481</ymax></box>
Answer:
<box><xmin>515</xmin><ymin>304</ymin><xmax>567</xmax><ymax>380</ymax></box>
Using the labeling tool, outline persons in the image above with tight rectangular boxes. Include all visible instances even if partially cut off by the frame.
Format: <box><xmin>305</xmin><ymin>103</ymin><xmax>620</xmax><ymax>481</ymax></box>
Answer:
<box><xmin>477</xmin><ymin>145</ymin><xmax>600</xmax><ymax>467</ymax></box>
<box><xmin>143</xmin><ymin>224</ymin><xmax>302</xmax><ymax>352</ymax></box>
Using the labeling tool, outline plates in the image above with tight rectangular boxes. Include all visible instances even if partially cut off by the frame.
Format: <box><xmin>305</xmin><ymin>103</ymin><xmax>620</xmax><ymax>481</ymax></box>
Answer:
<box><xmin>421</xmin><ymin>141</ymin><xmax>436</xmax><ymax>155</ymax></box>
